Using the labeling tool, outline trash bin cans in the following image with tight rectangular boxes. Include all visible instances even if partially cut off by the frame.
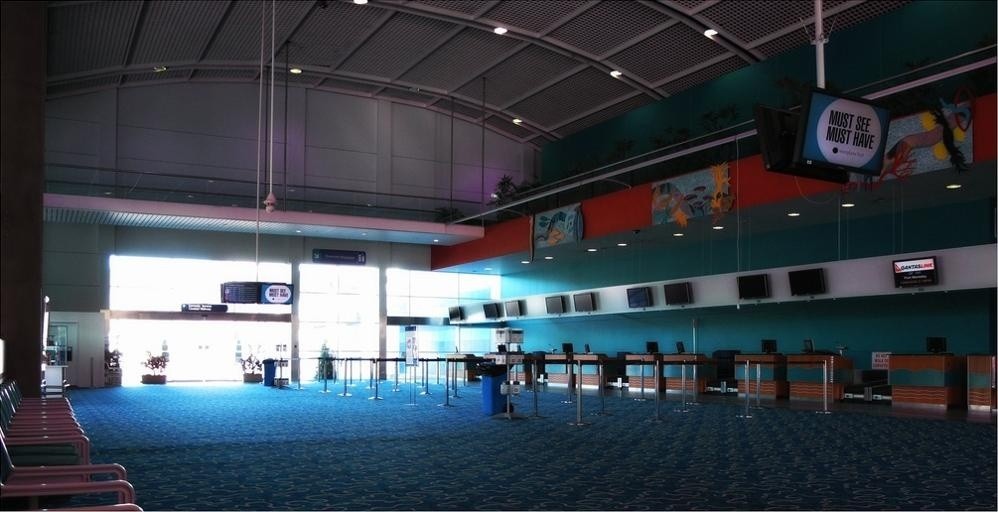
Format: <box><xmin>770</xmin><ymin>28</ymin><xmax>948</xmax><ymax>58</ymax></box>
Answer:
<box><xmin>482</xmin><ymin>362</ymin><xmax>514</xmax><ymax>416</ymax></box>
<box><xmin>262</xmin><ymin>359</ymin><xmax>278</xmax><ymax>386</ymax></box>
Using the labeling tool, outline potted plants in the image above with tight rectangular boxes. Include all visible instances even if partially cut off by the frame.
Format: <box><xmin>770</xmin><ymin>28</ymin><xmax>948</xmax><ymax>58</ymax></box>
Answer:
<box><xmin>314</xmin><ymin>343</ymin><xmax>333</xmax><ymax>380</ymax></box>
<box><xmin>141</xmin><ymin>355</ymin><xmax>169</xmax><ymax>384</ymax></box>
<box><xmin>241</xmin><ymin>354</ymin><xmax>262</xmax><ymax>382</ymax></box>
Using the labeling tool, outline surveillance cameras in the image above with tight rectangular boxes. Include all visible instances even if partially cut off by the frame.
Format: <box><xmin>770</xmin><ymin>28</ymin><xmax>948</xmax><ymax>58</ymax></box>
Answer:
<box><xmin>631</xmin><ymin>229</ymin><xmax>639</xmax><ymax>235</ymax></box>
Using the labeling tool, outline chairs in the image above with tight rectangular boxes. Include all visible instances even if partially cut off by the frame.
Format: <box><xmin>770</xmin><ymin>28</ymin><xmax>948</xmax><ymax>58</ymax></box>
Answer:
<box><xmin>0</xmin><ymin>371</ymin><xmax>143</xmax><ymax>511</ymax></box>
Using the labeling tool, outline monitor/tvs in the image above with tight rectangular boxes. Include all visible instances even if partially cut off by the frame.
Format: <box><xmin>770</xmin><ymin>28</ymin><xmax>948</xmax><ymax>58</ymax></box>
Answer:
<box><xmin>892</xmin><ymin>255</ymin><xmax>939</xmax><ymax>288</ymax></box>
<box><xmin>752</xmin><ymin>88</ymin><xmax>895</xmax><ymax>185</ymax></box>
<box><xmin>498</xmin><ymin>345</ymin><xmax>506</xmax><ymax>353</ymax></box>
<box><xmin>803</xmin><ymin>339</ymin><xmax>812</xmax><ymax>354</ymax></box>
<box><xmin>788</xmin><ymin>267</ymin><xmax>826</xmax><ymax>297</ymax></box>
<box><xmin>736</xmin><ymin>274</ymin><xmax>769</xmax><ymax>300</ymax></box>
<box><xmin>560</xmin><ymin>341</ymin><xmax>686</xmax><ymax>356</ymax></box>
<box><xmin>223</xmin><ymin>283</ymin><xmax>259</xmax><ymax>304</ymax></box>
<box><xmin>925</xmin><ymin>337</ymin><xmax>948</xmax><ymax>352</ymax></box>
<box><xmin>447</xmin><ymin>281</ymin><xmax>694</xmax><ymax>322</ymax></box>
<box><xmin>259</xmin><ymin>283</ymin><xmax>294</xmax><ymax>304</ymax></box>
<box><xmin>762</xmin><ymin>340</ymin><xmax>777</xmax><ymax>353</ymax></box>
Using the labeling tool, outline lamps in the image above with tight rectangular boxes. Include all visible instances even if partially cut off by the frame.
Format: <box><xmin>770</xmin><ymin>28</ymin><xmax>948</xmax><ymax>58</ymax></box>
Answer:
<box><xmin>262</xmin><ymin>1</ymin><xmax>277</xmax><ymax>214</ymax></box>
